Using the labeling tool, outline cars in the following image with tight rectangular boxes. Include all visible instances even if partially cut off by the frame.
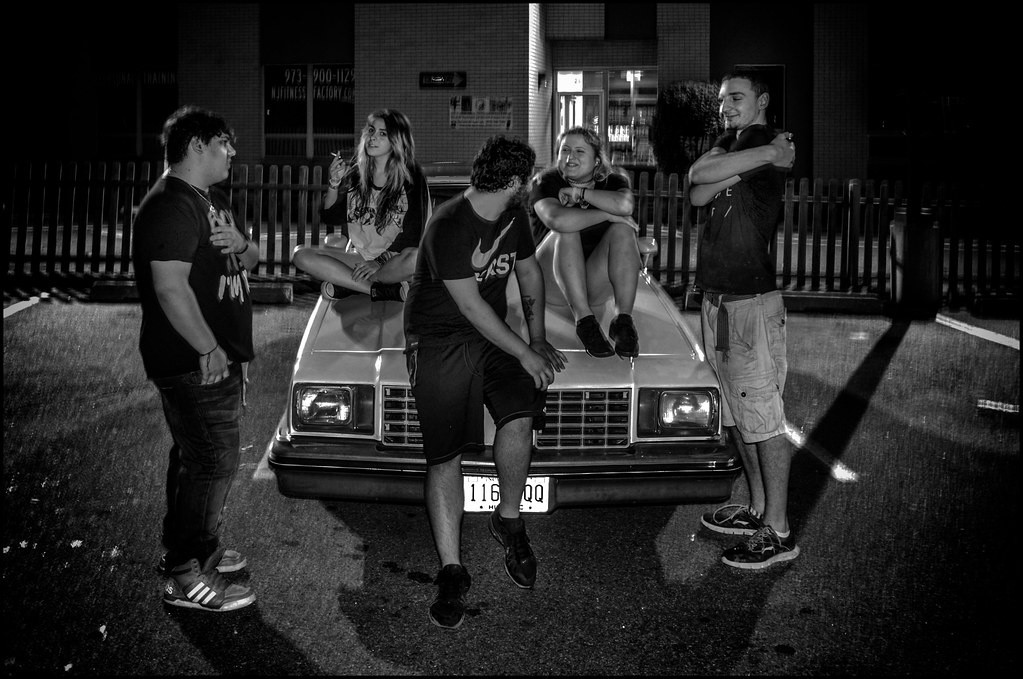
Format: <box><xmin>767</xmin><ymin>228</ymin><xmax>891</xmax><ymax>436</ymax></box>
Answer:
<box><xmin>266</xmin><ymin>233</ymin><xmax>744</xmax><ymax>517</ymax></box>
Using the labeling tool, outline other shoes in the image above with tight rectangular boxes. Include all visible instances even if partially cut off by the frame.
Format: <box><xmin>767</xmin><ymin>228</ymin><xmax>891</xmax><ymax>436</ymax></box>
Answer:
<box><xmin>576</xmin><ymin>316</ymin><xmax>615</xmax><ymax>358</ymax></box>
<box><xmin>610</xmin><ymin>314</ymin><xmax>640</xmax><ymax>356</ymax></box>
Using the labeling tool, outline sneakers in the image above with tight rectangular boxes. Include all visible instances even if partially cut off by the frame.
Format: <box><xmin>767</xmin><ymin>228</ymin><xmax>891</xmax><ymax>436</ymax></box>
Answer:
<box><xmin>160</xmin><ymin>549</ymin><xmax>247</xmax><ymax>572</ymax></box>
<box><xmin>722</xmin><ymin>524</ymin><xmax>800</xmax><ymax>569</ymax></box>
<box><xmin>700</xmin><ymin>504</ymin><xmax>764</xmax><ymax>535</ymax></box>
<box><xmin>488</xmin><ymin>505</ymin><xmax>536</xmax><ymax>588</ymax></box>
<box><xmin>428</xmin><ymin>564</ymin><xmax>472</xmax><ymax>629</ymax></box>
<box><xmin>164</xmin><ymin>546</ymin><xmax>257</xmax><ymax>612</ymax></box>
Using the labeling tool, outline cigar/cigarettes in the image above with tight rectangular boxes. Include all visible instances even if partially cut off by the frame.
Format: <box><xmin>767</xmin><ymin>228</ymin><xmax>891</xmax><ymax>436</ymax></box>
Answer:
<box><xmin>330</xmin><ymin>152</ymin><xmax>341</xmax><ymax>158</ymax></box>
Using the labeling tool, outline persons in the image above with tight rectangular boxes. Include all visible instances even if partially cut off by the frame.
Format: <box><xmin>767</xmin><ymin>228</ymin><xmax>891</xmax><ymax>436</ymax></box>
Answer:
<box><xmin>528</xmin><ymin>127</ymin><xmax>641</xmax><ymax>356</ymax></box>
<box><xmin>291</xmin><ymin>109</ymin><xmax>432</xmax><ymax>302</ymax></box>
<box><xmin>131</xmin><ymin>106</ymin><xmax>259</xmax><ymax>611</ymax></box>
<box><xmin>402</xmin><ymin>137</ymin><xmax>568</xmax><ymax>628</ymax></box>
<box><xmin>685</xmin><ymin>70</ymin><xmax>799</xmax><ymax>568</ymax></box>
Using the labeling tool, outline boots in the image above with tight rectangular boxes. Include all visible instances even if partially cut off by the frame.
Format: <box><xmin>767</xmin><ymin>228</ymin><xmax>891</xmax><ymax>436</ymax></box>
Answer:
<box><xmin>320</xmin><ymin>281</ymin><xmax>361</xmax><ymax>300</ymax></box>
<box><xmin>370</xmin><ymin>280</ymin><xmax>410</xmax><ymax>302</ymax></box>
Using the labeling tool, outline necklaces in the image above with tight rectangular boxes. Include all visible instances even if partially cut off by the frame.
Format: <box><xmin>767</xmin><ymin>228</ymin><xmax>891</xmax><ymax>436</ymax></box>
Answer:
<box><xmin>189</xmin><ymin>184</ymin><xmax>215</xmax><ymax>212</ymax></box>
<box><xmin>568</xmin><ymin>179</ymin><xmax>593</xmax><ymax>185</ymax></box>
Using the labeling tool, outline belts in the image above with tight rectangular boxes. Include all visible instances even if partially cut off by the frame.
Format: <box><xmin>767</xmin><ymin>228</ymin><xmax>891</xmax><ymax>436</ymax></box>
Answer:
<box><xmin>703</xmin><ymin>291</ymin><xmax>757</xmax><ymax>350</ymax></box>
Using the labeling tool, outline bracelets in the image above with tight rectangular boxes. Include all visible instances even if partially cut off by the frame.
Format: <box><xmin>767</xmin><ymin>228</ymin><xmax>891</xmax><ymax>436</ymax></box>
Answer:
<box><xmin>328</xmin><ymin>179</ymin><xmax>342</xmax><ymax>189</ymax></box>
<box><xmin>235</xmin><ymin>233</ymin><xmax>249</xmax><ymax>254</ymax></box>
<box><xmin>580</xmin><ymin>187</ymin><xmax>588</xmax><ymax>204</ymax></box>
<box><xmin>200</xmin><ymin>344</ymin><xmax>218</xmax><ymax>367</ymax></box>
